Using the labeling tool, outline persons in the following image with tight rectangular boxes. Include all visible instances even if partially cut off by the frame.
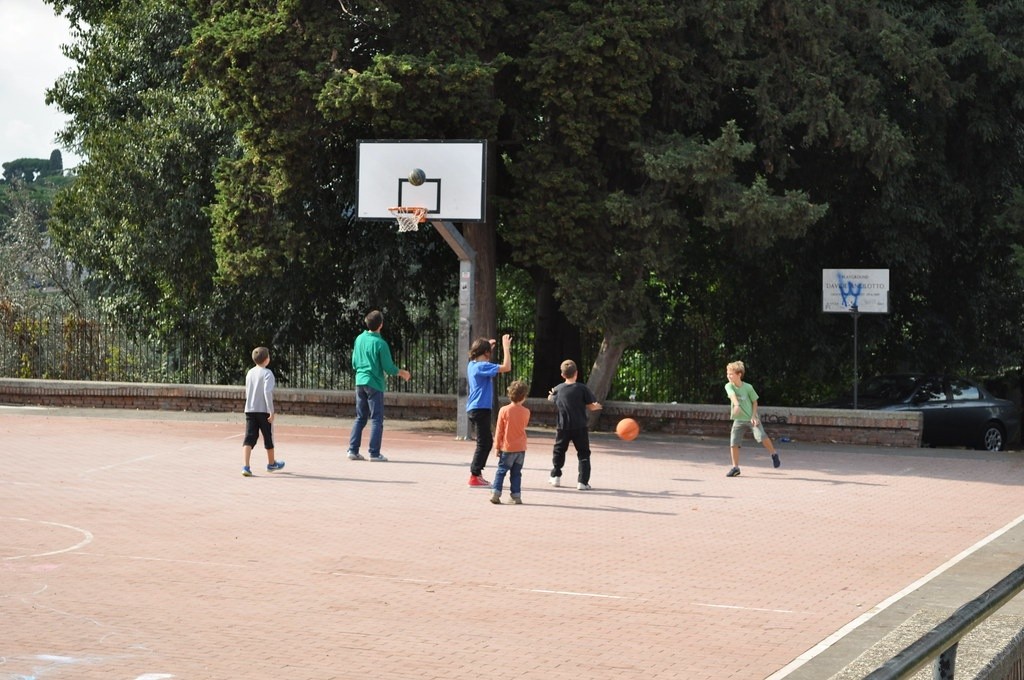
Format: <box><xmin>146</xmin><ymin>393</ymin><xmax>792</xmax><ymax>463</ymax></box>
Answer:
<box><xmin>346</xmin><ymin>309</ymin><xmax>410</xmax><ymax>463</ymax></box>
<box><xmin>464</xmin><ymin>333</ymin><xmax>513</xmax><ymax>488</ymax></box>
<box><xmin>488</xmin><ymin>379</ymin><xmax>531</xmax><ymax>505</ymax></box>
<box><xmin>241</xmin><ymin>347</ymin><xmax>286</xmax><ymax>478</ymax></box>
<box><xmin>546</xmin><ymin>360</ymin><xmax>605</xmax><ymax>491</ymax></box>
<box><xmin>724</xmin><ymin>360</ymin><xmax>781</xmax><ymax>478</ymax></box>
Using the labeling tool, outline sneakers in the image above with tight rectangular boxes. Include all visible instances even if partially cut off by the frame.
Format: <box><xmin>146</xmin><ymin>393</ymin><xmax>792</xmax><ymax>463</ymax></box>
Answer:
<box><xmin>549</xmin><ymin>476</ymin><xmax>591</xmax><ymax>490</ymax></box>
<box><xmin>726</xmin><ymin>467</ymin><xmax>740</xmax><ymax>477</ymax></box>
<box><xmin>267</xmin><ymin>460</ymin><xmax>285</xmax><ymax>472</ymax></box>
<box><xmin>490</xmin><ymin>491</ymin><xmax>523</xmax><ymax>504</ymax></box>
<box><xmin>349</xmin><ymin>451</ymin><xmax>364</xmax><ymax>461</ymax></box>
<box><xmin>242</xmin><ymin>466</ymin><xmax>252</xmax><ymax>477</ymax></box>
<box><xmin>369</xmin><ymin>454</ymin><xmax>387</xmax><ymax>462</ymax></box>
<box><xmin>468</xmin><ymin>473</ymin><xmax>492</xmax><ymax>487</ymax></box>
<box><xmin>771</xmin><ymin>453</ymin><xmax>780</xmax><ymax>468</ymax></box>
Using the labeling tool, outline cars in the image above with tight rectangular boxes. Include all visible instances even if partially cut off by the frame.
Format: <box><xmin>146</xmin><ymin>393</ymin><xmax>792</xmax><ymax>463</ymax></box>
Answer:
<box><xmin>798</xmin><ymin>370</ymin><xmax>1020</xmax><ymax>453</ymax></box>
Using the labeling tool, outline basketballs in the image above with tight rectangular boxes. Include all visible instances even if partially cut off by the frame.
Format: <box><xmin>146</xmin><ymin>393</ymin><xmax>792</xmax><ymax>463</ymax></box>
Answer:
<box><xmin>616</xmin><ymin>418</ymin><xmax>640</xmax><ymax>441</ymax></box>
<box><xmin>408</xmin><ymin>168</ymin><xmax>426</xmax><ymax>187</ymax></box>
